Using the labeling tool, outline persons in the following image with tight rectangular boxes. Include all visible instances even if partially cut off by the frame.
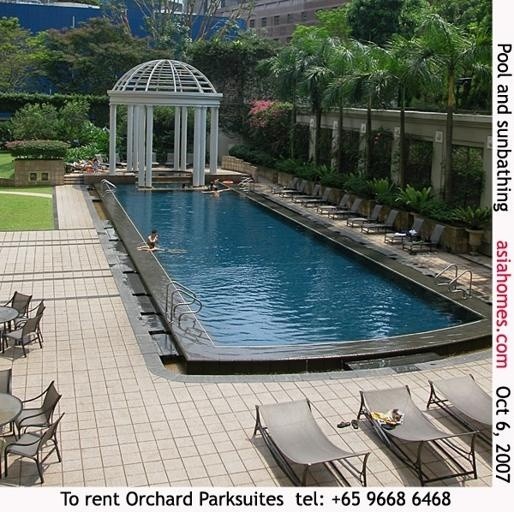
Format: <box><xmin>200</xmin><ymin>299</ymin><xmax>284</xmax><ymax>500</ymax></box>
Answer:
<box><xmin>201</xmin><ymin>179</ymin><xmax>232</xmax><ymax>198</ymax></box>
<box><xmin>75</xmin><ymin>158</ymin><xmax>103</xmax><ymax>174</ymax></box>
<box><xmin>137</xmin><ymin>230</ymin><xmax>189</xmax><ymax>255</ymax></box>
<box><xmin>372</xmin><ymin>408</ymin><xmax>401</xmax><ymax>427</ymax></box>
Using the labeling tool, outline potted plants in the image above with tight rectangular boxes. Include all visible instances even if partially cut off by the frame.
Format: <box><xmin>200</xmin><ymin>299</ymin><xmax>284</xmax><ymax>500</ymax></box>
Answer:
<box><xmin>455</xmin><ymin>203</ymin><xmax>492</xmax><ymax>257</ymax></box>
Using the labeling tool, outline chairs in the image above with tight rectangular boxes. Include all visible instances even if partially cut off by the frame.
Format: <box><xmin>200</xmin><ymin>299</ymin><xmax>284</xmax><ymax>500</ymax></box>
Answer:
<box><xmin>427</xmin><ymin>375</ymin><xmax>493</xmax><ymax>451</ymax></box>
<box><xmin>71</xmin><ymin>150</ymin><xmax>196</xmax><ymax>170</ymax></box>
<box><xmin>274</xmin><ymin>176</ymin><xmax>446</xmax><ymax>256</ymax></box>
<box><xmin>250</xmin><ymin>398</ymin><xmax>370</xmax><ymax>487</ymax></box>
<box><xmin>0</xmin><ymin>369</ymin><xmax>67</xmax><ymax>484</ymax></box>
<box><xmin>0</xmin><ymin>290</ymin><xmax>52</xmax><ymax>361</ymax></box>
<box><xmin>359</xmin><ymin>385</ymin><xmax>483</xmax><ymax>486</ymax></box>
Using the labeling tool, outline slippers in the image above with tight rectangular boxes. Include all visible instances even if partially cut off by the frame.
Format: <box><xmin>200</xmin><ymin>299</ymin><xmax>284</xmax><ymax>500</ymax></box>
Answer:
<box><xmin>337</xmin><ymin>421</ymin><xmax>350</xmax><ymax>428</ymax></box>
<box><xmin>351</xmin><ymin>419</ymin><xmax>359</xmax><ymax>430</ymax></box>
